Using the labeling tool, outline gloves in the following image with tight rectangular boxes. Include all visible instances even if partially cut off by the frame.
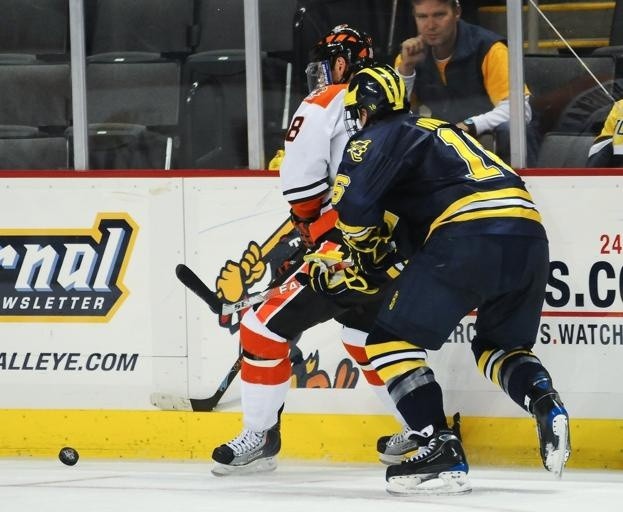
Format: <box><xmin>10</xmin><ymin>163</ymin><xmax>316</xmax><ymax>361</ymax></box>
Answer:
<box><xmin>334</xmin><ymin>217</ymin><xmax>397</xmax><ymax>275</ymax></box>
<box><xmin>302</xmin><ymin>251</ymin><xmax>369</xmax><ymax>298</ymax></box>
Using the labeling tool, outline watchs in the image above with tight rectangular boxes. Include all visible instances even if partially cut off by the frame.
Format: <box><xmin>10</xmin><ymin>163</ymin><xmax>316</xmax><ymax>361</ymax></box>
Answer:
<box><xmin>462</xmin><ymin>117</ymin><xmax>476</xmax><ymax>137</ymax></box>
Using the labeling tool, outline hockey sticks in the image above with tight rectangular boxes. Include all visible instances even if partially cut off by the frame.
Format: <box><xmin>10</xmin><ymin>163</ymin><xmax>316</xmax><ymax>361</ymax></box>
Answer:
<box><xmin>175</xmin><ymin>256</ymin><xmax>352</xmax><ymax>316</ymax></box>
<box><xmin>149</xmin><ymin>352</ymin><xmax>241</xmax><ymax>411</ymax></box>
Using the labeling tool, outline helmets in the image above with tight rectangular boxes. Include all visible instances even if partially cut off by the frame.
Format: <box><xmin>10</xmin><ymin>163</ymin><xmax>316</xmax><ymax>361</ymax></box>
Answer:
<box><xmin>342</xmin><ymin>63</ymin><xmax>408</xmax><ymax>138</ymax></box>
<box><xmin>303</xmin><ymin>23</ymin><xmax>377</xmax><ymax>93</ymax></box>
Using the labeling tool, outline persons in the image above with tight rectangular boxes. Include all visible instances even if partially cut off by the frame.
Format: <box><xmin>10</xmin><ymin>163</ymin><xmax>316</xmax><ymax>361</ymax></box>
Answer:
<box><xmin>588</xmin><ymin>98</ymin><xmax>623</xmax><ymax>167</ymax></box>
<box><xmin>394</xmin><ymin>0</ymin><xmax>544</xmax><ymax>168</ymax></box>
<box><xmin>208</xmin><ymin>22</ymin><xmax>446</xmax><ymax>478</ymax></box>
<box><xmin>329</xmin><ymin>61</ymin><xmax>572</xmax><ymax>497</ymax></box>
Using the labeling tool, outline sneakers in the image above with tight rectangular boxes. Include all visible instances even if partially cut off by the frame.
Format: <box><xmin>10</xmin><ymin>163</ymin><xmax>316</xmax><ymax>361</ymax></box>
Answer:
<box><xmin>524</xmin><ymin>370</ymin><xmax>571</xmax><ymax>474</ymax></box>
<box><xmin>211</xmin><ymin>402</ymin><xmax>284</xmax><ymax>467</ymax></box>
<box><xmin>385</xmin><ymin>412</ymin><xmax>467</xmax><ymax>487</ymax></box>
<box><xmin>376</xmin><ymin>426</ymin><xmax>419</xmax><ymax>456</ymax></box>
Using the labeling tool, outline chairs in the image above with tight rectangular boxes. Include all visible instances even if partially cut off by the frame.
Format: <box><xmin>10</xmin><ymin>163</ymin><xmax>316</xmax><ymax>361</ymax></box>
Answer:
<box><xmin>519</xmin><ymin>52</ymin><xmax>611</xmax><ymax>133</ymax></box>
<box><xmin>184</xmin><ymin>50</ymin><xmax>271</xmax><ymax>174</ymax></box>
<box><xmin>460</xmin><ymin>5</ymin><xmax>507</xmax><ymax>40</ymax></box>
<box><xmin>55</xmin><ymin>121</ymin><xmax>150</xmax><ymax>176</ymax></box>
<box><xmin>85</xmin><ymin>48</ymin><xmax>181</xmax><ymax>130</ymax></box>
<box><xmin>0</xmin><ymin>123</ymin><xmax>59</xmax><ymax>172</ymax></box>
<box><xmin>0</xmin><ymin>56</ymin><xmax>71</xmax><ymax>126</ymax></box>
<box><xmin>194</xmin><ymin>0</ymin><xmax>296</xmax><ymax>53</ymax></box>
<box><xmin>0</xmin><ymin>0</ymin><xmax>72</xmax><ymax>54</ymax></box>
<box><xmin>535</xmin><ymin>132</ymin><xmax>617</xmax><ymax>172</ymax></box>
